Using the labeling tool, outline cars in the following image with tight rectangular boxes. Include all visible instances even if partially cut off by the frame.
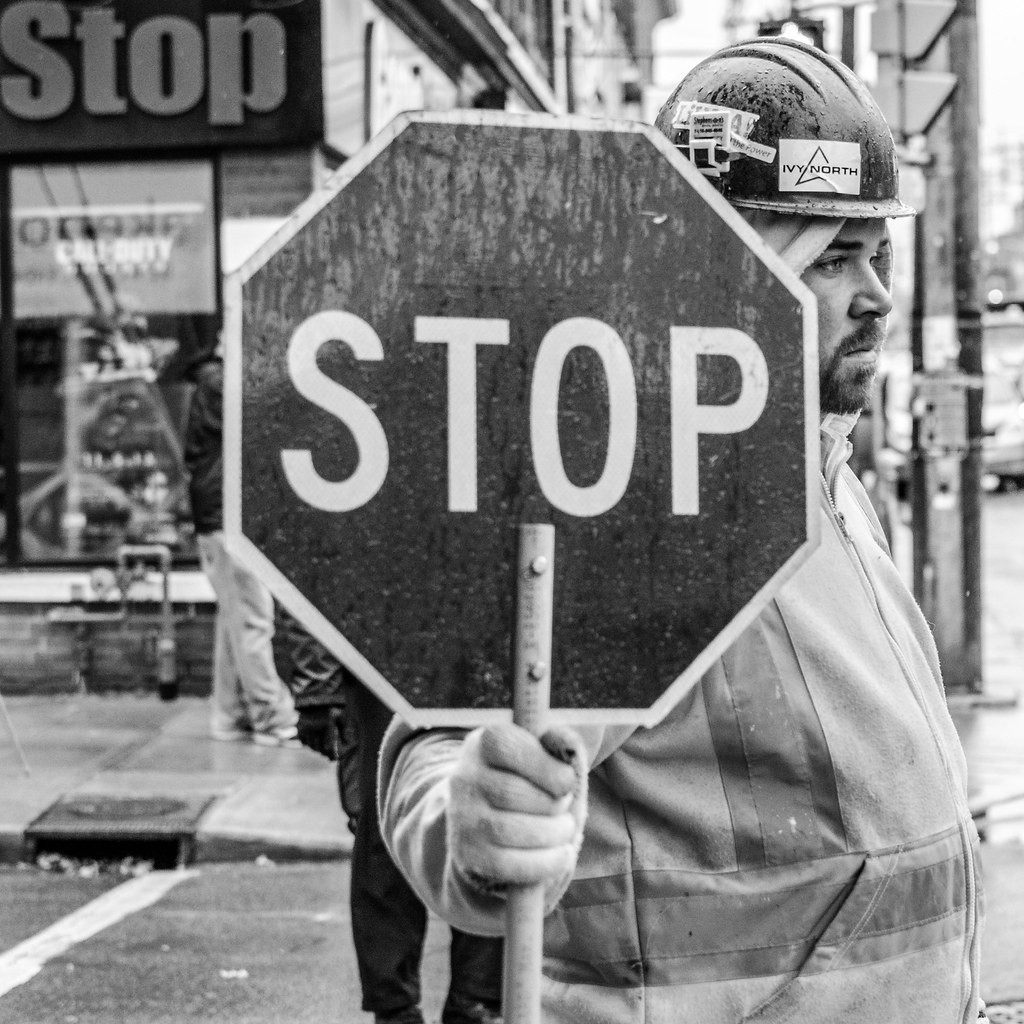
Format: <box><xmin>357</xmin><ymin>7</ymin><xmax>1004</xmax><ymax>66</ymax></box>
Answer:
<box><xmin>867</xmin><ymin>315</ymin><xmax>1023</xmax><ymax>502</ymax></box>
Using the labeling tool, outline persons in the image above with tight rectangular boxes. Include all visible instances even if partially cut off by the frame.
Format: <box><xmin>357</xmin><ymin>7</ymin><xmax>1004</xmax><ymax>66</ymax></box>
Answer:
<box><xmin>379</xmin><ymin>37</ymin><xmax>984</xmax><ymax>1024</ymax></box>
<box><xmin>186</xmin><ymin>352</ymin><xmax>306</xmax><ymax>746</ymax></box>
<box><xmin>274</xmin><ymin>600</ymin><xmax>502</xmax><ymax>1024</ymax></box>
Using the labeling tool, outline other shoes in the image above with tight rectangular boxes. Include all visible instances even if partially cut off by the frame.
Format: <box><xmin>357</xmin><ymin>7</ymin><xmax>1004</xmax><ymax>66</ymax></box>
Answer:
<box><xmin>212</xmin><ymin>724</ymin><xmax>252</xmax><ymax>741</ymax></box>
<box><xmin>253</xmin><ymin>729</ymin><xmax>302</xmax><ymax>749</ymax></box>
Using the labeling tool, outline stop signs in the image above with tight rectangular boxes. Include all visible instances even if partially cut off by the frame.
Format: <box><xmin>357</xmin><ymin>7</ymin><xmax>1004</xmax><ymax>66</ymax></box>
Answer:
<box><xmin>222</xmin><ymin>108</ymin><xmax>820</xmax><ymax>731</ymax></box>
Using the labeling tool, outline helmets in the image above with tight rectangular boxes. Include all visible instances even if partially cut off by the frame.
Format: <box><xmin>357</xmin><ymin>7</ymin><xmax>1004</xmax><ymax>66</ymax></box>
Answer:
<box><xmin>655</xmin><ymin>36</ymin><xmax>917</xmax><ymax>218</ymax></box>
